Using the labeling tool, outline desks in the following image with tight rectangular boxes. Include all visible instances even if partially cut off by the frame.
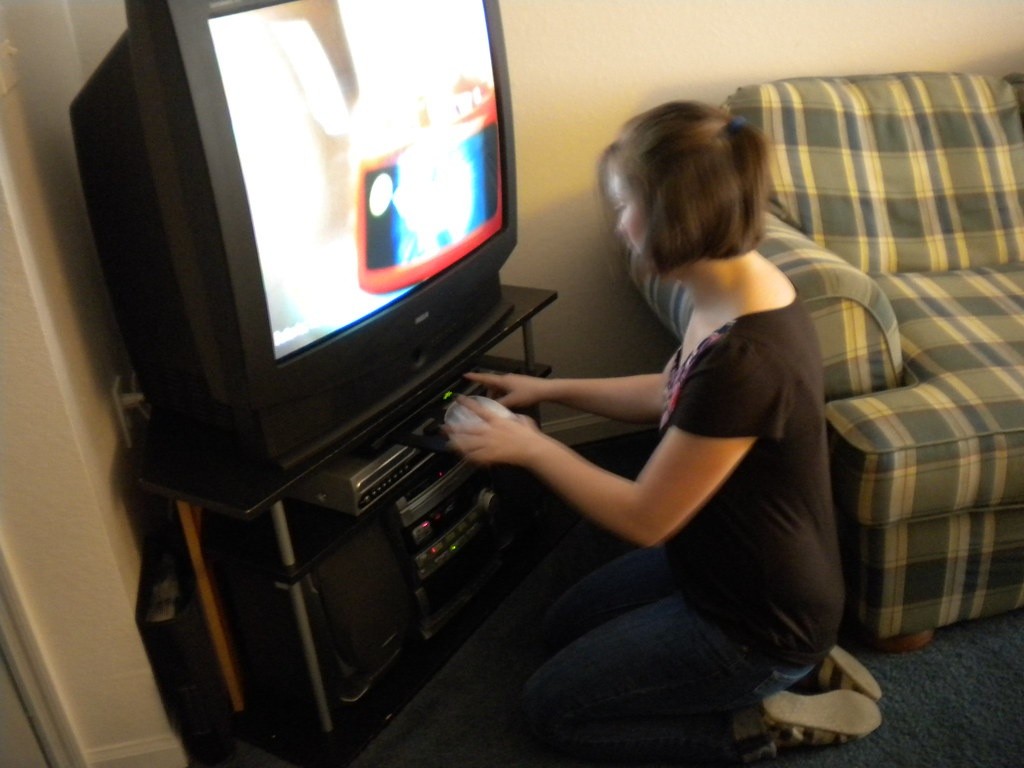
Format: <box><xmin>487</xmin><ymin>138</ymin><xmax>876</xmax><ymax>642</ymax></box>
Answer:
<box><xmin>117</xmin><ymin>282</ymin><xmax>559</xmax><ymax>767</ymax></box>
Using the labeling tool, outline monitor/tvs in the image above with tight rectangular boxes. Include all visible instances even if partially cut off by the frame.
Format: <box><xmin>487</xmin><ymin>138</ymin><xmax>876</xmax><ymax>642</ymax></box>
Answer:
<box><xmin>65</xmin><ymin>0</ymin><xmax>522</xmax><ymax>475</ymax></box>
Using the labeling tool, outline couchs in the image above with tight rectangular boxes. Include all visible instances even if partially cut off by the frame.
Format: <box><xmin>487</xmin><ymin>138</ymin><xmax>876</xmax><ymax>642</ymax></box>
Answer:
<box><xmin>625</xmin><ymin>66</ymin><xmax>1024</xmax><ymax>652</ymax></box>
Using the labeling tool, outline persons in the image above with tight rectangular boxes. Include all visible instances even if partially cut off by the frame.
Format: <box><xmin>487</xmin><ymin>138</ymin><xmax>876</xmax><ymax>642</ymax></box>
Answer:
<box><xmin>442</xmin><ymin>101</ymin><xmax>883</xmax><ymax>768</ymax></box>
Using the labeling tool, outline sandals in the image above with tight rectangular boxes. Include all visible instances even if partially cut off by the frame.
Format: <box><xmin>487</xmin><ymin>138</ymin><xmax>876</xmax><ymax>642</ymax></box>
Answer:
<box><xmin>805</xmin><ymin>646</ymin><xmax>883</xmax><ymax>705</ymax></box>
<box><xmin>757</xmin><ymin>690</ymin><xmax>882</xmax><ymax>747</ymax></box>
